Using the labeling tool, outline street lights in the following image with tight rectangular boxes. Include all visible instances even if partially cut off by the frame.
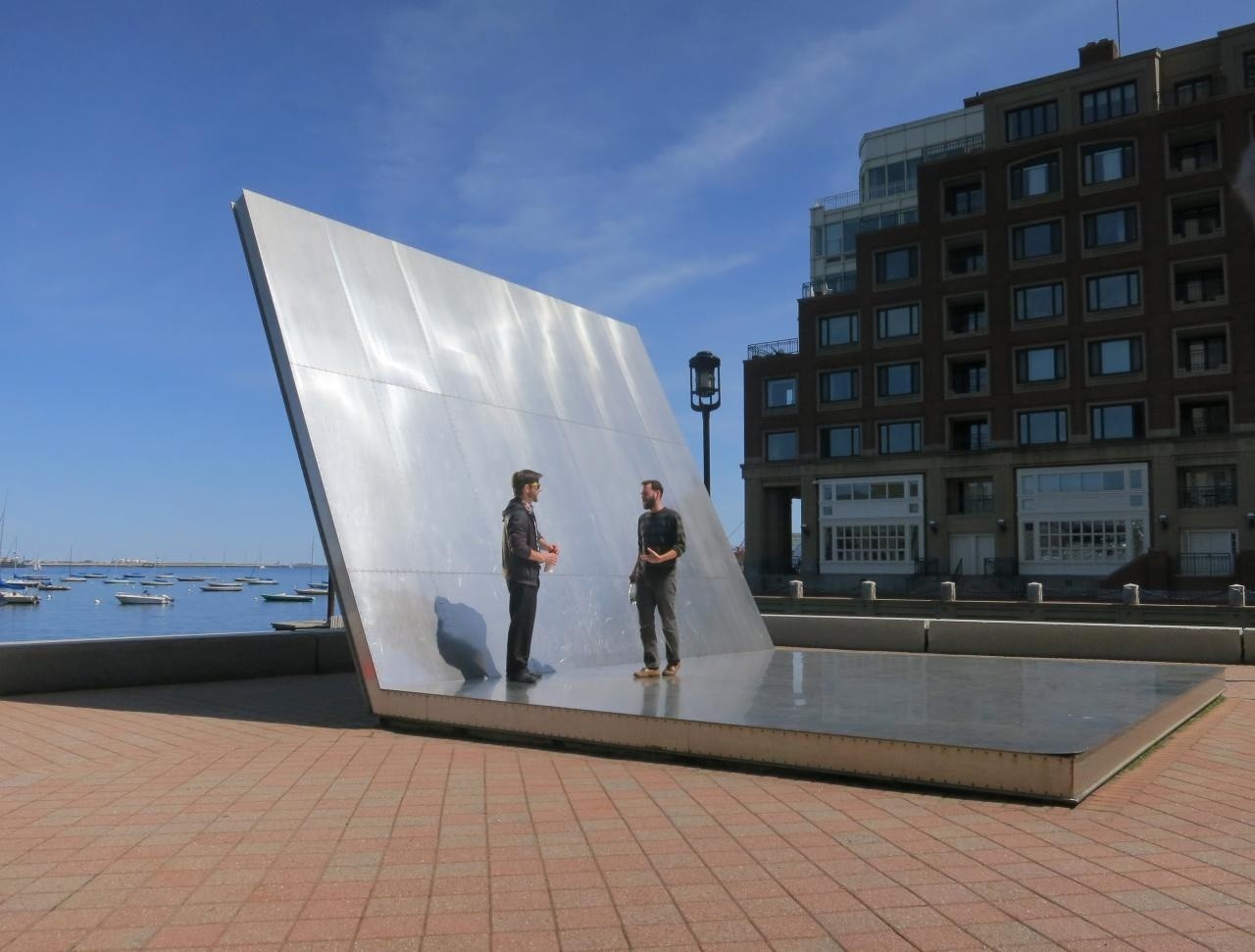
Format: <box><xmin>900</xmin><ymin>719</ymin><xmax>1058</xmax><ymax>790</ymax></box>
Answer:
<box><xmin>688</xmin><ymin>350</ymin><xmax>721</xmax><ymax>499</ymax></box>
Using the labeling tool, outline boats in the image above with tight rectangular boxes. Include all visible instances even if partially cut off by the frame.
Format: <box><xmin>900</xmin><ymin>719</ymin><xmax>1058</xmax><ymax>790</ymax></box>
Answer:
<box><xmin>198</xmin><ymin>586</ymin><xmax>244</xmax><ymax>592</ymax></box>
<box><xmin>234</xmin><ymin>552</ymin><xmax>281</xmax><ymax>585</ymax></box>
<box><xmin>114</xmin><ymin>590</ymin><xmax>175</xmax><ymax>605</ymax></box>
<box><xmin>260</xmin><ymin>593</ymin><xmax>316</xmax><ymax>603</ymax></box>
<box><xmin>307</xmin><ymin>535</ymin><xmax>329</xmax><ymax>589</ymax></box>
<box><xmin>294</xmin><ymin>588</ymin><xmax>329</xmax><ymax>596</ymax></box>
<box><xmin>0</xmin><ymin>534</ymin><xmax>54</xmax><ymax>589</ymax></box>
<box><xmin>206</xmin><ymin>581</ymin><xmax>243</xmax><ymax>587</ymax></box>
<box><xmin>38</xmin><ymin>583</ymin><xmax>71</xmax><ymax>591</ymax></box>
<box><xmin>177</xmin><ymin>576</ymin><xmax>205</xmax><ymax>582</ymax></box>
<box><xmin>0</xmin><ymin>591</ymin><xmax>42</xmax><ymax>605</ymax></box>
<box><xmin>57</xmin><ymin>544</ymin><xmax>177</xmax><ymax>586</ymax></box>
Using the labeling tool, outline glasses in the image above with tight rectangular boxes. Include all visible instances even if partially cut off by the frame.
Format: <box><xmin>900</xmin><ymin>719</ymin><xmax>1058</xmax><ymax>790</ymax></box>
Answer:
<box><xmin>529</xmin><ymin>483</ymin><xmax>541</xmax><ymax>488</ymax></box>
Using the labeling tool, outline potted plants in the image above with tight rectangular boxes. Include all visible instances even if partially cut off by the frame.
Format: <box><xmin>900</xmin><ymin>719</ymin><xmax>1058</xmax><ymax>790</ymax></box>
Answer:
<box><xmin>752</xmin><ymin>356</ymin><xmax>761</xmax><ymax>360</ymax></box>
<box><xmin>766</xmin><ymin>354</ymin><xmax>776</xmax><ymax>361</ymax></box>
<box><xmin>776</xmin><ymin>352</ymin><xmax>785</xmax><ymax>361</ymax></box>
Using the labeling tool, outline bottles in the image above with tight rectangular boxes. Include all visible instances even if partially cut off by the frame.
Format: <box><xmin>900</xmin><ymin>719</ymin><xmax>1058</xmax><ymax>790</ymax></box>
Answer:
<box><xmin>627</xmin><ymin>583</ymin><xmax>637</xmax><ymax>605</ymax></box>
<box><xmin>544</xmin><ymin>545</ymin><xmax>557</xmax><ymax>573</ymax></box>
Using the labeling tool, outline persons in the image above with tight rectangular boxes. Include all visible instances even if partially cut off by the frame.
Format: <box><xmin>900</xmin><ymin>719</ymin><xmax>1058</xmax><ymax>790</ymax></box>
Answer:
<box><xmin>500</xmin><ymin>470</ymin><xmax>562</xmax><ymax>684</ymax></box>
<box><xmin>627</xmin><ymin>479</ymin><xmax>687</xmax><ymax>678</ymax></box>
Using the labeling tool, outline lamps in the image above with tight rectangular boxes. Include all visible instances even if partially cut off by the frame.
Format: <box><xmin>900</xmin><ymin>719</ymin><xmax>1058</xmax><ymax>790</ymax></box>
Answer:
<box><xmin>1157</xmin><ymin>514</ymin><xmax>1169</xmax><ymax>531</ymax></box>
<box><xmin>1243</xmin><ymin>510</ymin><xmax>1255</xmax><ymax>528</ymax></box>
<box><xmin>928</xmin><ymin>519</ymin><xmax>939</xmax><ymax>534</ymax></box>
<box><xmin>801</xmin><ymin>524</ymin><xmax>809</xmax><ymax>536</ymax></box>
<box><xmin>996</xmin><ymin>517</ymin><xmax>1007</xmax><ymax>533</ymax></box>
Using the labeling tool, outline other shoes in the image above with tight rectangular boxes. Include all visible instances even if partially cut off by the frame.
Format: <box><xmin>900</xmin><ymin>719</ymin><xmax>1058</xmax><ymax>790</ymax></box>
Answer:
<box><xmin>525</xmin><ymin>669</ymin><xmax>542</xmax><ymax>678</ymax></box>
<box><xmin>634</xmin><ymin>667</ymin><xmax>660</xmax><ymax>676</ymax></box>
<box><xmin>506</xmin><ymin>671</ymin><xmax>536</xmax><ymax>683</ymax></box>
<box><xmin>663</xmin><ymin>661</ymin><xmax>679</xmax><ymax>675</ymax></box>
